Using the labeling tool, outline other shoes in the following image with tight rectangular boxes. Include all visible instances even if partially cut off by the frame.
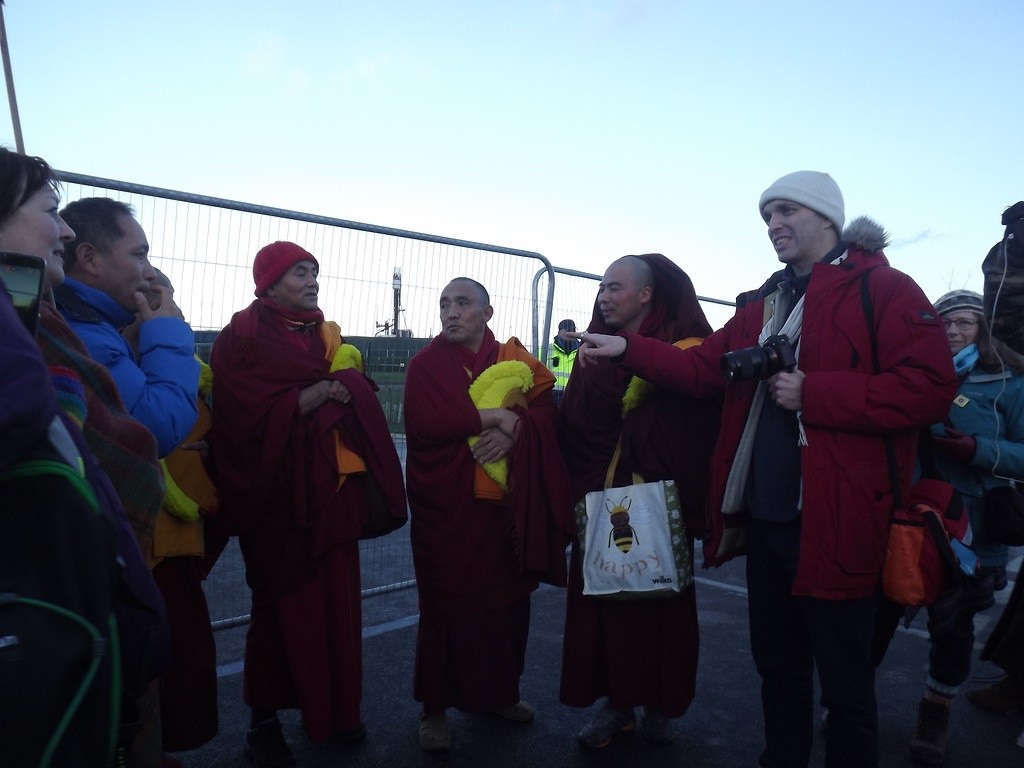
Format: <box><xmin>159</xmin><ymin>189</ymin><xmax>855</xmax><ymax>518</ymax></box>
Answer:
<box><xmin>985</xmin><ymin>673</ymin><xmax>1023</xmax><ymax>712</ymax></box>
<box><xmin>642</xmin><ymin>704</ymin><xmax>668</xmax><ymax>723</ymax></box>
<box><xmin>161</xmin><ymin>753</ymin><xmax>185</xmax><ymax>768</ymax></box>
<box><xmin>494</xmin><ymin>699</ymin><xmax>535</xmax><ymax>722</ymax></box>
<box><xmin>245</xmin><ymin>718</ymin><xmax>299</xmax><ymax>768</ymax></box>
<box><xmin>577</xmin><ymin>702</ymin><xmax>637</xmax><ymax>748</ymax></box>
<box><xmin>335</xmin><ymin>722</ymin><xmax>368</xmax><ymax>740</ymax></box>
<box><xmin>418</xmin><ymin>706</ymin><xmax>452</xmax><ymax>751</ymax></box>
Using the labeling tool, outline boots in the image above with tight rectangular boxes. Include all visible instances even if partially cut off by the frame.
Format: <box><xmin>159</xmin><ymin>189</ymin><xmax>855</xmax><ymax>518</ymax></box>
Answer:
<box><xmin>818</xmin><ymin>708</ymin><xmax>833</xmax><ymax>733</ymax></box>
<box><xmin>911</xmin><ymin>694</ymin><xmax>956</xmax><ymax>763</ymax></box>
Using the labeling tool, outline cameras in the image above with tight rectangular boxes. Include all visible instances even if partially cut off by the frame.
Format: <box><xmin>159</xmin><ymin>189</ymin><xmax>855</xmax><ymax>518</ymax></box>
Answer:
<box><xmin>720</xmin><ymin>334</ymin><xmax>797</xmax><ymax>386</ymax></box>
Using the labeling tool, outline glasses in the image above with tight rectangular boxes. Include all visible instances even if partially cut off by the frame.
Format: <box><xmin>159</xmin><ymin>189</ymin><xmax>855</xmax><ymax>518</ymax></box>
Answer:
<box><xmin>941</xmin><ymin>316</ymin><xmax>979</xmax><ymax>330</ymax></box>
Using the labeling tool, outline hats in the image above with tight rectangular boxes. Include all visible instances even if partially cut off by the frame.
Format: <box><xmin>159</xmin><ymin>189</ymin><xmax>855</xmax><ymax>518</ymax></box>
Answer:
<box><xmin>252</xmin><ymin>241</ymin><xmax>318</xmax><ymax>298</ymax></box>
<box><xmin>758</xmin><ymin>170</ymin><xmax>845</xmax><ymax>239</ymax></box>
<box><xmin>931</xmin><ymin>290</ymin><xmax>985</xmax><ymax>319</ymax></box>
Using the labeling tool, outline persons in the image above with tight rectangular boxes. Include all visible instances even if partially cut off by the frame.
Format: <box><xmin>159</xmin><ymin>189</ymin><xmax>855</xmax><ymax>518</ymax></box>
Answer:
<box><xmin>212</xmin><ymin>243</ymin><xmax>368</xmax><ymax>768</ymax></box>
<box><xmin>127</xmin><ymin>269</ymin><xmax>227</xmax><ymax>768</ymax></box>
<box><xmin>538</xmin><ymin>319</ymin><xmax>580</xmax><ymax>406</ymax></box>
<box><xmin>0</xmin><ymin>145</ymin><xmax>165</xmax><ymax>768</ymax></box>
<box><xmin>820</xmin><ymin>200</ymin><xmax>1024</xmax><ymax>751</ymax></box>
<box><xmin>565</xmin><ymin>171</ymin><xmax>958</xmax><ymax>768</ymax></box>
<box><xmin>405</xmin><ymin>277</ymin><xmax>537</xmax><ymax>752</ymax></box>
<box><xmin>57</xmin><ymin>194</ymin><xmax>198</xmax><ymax>451</ymax></box>
<box><xmin>556</xmin><ymin>253</ymin><xmax>715</xmax><ymax>749</ymax></box>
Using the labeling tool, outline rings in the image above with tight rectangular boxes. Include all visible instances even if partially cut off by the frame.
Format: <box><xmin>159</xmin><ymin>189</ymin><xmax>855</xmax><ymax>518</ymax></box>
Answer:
<box><xmin>581</xmin><ymin>331</ymin><xmax>588</xmax><ymax>341</ymax></box>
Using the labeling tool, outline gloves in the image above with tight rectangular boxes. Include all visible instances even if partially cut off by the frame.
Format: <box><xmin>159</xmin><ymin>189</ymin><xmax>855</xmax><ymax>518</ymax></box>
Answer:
<box><xmin>933</xmin><ymin>427</ymin><xmax>976</xmax><ymax>462</ymax></box>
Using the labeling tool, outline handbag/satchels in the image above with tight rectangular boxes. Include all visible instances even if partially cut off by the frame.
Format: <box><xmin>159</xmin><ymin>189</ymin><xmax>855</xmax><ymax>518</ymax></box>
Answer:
<box><xmin>983</xmin><ymin>484</ymin><xmax>1024</xmax><ymax>547</ymax></box>
<box><xmin>574</xmin><ymin>439</ymin><xmax>694</xmax><ymax>603</ymax></box>
<box><xmin>0</xmin><ymin>415</ymin><xmax>143</xmax><ymax>768</ymax></box>
<box><xmin>883</xmin><ymin>478</ymin><xmax>978</xmax><ymax>613</ymax></box>
